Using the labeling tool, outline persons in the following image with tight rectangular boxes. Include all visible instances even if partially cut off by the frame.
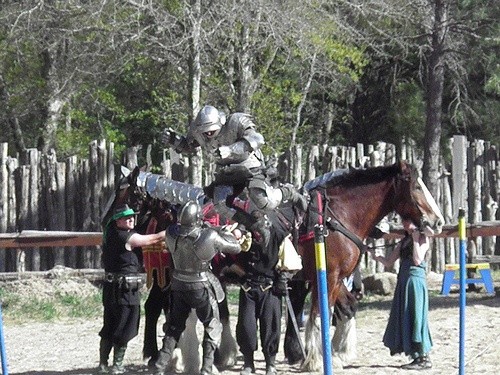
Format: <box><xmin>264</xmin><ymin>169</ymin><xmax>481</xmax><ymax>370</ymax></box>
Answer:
<box><xmin>162</xmin><ymin>104</ymin><xmax>307</xmax><ymax>375</ymax></box>
<box><xmin>351</xmin><ymin>219</ymin><xmax>434</xmax><ymax>370</ymax></box>
<box><xmin>98</xmin><ymin>202</ymin><xmax>166</xmax><ymax>375</ymax></box>
<box><xmin>154</xmin><ymin>200</ymin><xmax>242</xmax><ymax>375</ymax></box>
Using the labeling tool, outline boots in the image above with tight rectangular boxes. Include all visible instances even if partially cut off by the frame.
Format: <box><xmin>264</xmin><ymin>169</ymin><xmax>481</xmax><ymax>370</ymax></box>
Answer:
<box><xmin>279</xmin><ymin>181</ymin><xmax>308</xmax><ymax>213</ymax></box>
<box><xmin>98</xmin><ymin>336</ymin><xmax>114</xmax><ymax>373</ymax></box>
<box><xmin>265</xmin><ymin>356</ymin><xmax>277</xmax><ymax>375</ymax></box>
<box><xmin>240</xmin><ymin>355</ymin><xmax>255</xmax><ymax>375</ymax></box>
<box><xmin>110</xmin><ymin>344</ymin><xmax>127</xmax><ymax>374</ymax></box>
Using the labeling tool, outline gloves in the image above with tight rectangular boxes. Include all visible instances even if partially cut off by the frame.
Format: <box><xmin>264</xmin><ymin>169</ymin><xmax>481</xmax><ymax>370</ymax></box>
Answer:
<box><xmin>223</xmin><ymin>263</ymin><xmax>246</xmax><ymax>283</ymax></box>
<box><xmin>209</xmin><ymin>144</ymin><xmax>230</xmax><ymax>159</ymax></box>
<box><xmin>157</xmin><ymin>126</ymin><xmax>182</xmax><ymax>150</ymax></box>
<box><xmin>272</xmin><ymin>271</ymin><xmax>289</xmax><ymax>294</ymax></box>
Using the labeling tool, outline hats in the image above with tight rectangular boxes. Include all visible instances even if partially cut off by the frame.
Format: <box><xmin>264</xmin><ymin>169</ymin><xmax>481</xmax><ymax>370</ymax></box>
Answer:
<box><xmin>114</xmin><ymin>207</ymin><xmax>141</xmax><ymax>220</ymax></box>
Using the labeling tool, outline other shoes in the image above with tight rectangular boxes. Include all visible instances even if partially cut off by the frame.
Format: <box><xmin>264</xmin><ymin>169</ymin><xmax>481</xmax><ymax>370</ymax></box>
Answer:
<box><xmin>400</xmin><ymin>354</ymin><xmax>431</xmax><ymax>370</ymax></box>
<box><xmin>200</xmin><ymin>369</ymin><xmax>216</xmax><ymax>375</ymax></box>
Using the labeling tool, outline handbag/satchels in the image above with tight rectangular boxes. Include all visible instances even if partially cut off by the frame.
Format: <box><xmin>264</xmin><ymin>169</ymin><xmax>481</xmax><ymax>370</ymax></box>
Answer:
<box><xmin>124</xmin><ymin>275</ymin><xmax>143</xmax><ymax>304</ymax></box>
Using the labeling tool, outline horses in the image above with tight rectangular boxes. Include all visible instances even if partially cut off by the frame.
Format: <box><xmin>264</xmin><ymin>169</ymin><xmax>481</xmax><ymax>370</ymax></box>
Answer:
<box><xmin>99</xmin><ymin>158</ymin><xmax>445</xmax><ymax>375</ymax></box>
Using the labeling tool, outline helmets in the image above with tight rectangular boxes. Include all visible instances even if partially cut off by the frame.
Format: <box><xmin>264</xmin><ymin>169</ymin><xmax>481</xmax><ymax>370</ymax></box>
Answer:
<box><xmin>175</xmin><ymin>199</ymin><xmax>202</xmax><ymax>238</ymax></box>
<box><xmin>194</xmin><ymin>104</ymin><xmax>226</xmax><ymax>138</ymax></box>
